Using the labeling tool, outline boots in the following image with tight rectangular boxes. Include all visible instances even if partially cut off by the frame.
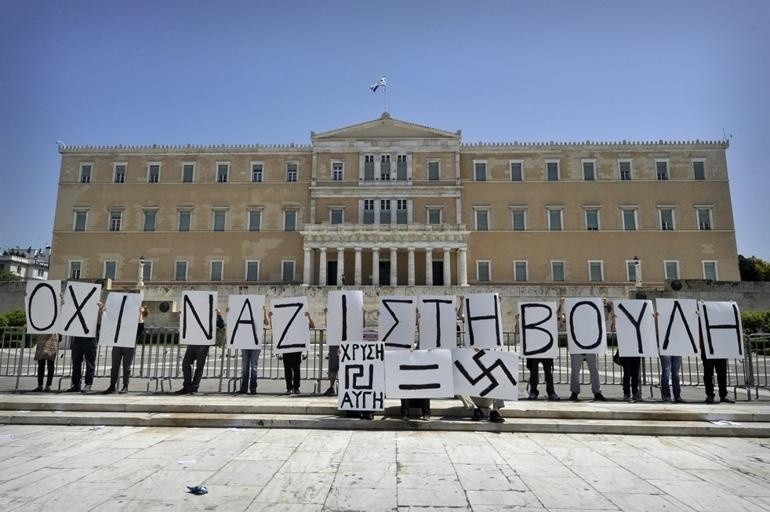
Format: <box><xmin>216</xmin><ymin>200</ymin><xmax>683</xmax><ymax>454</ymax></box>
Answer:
<box><xmin>471</xmin><ymin>408</ymin><xmax>483</xmax><ymax>420</ymax></box>
<box><xmin>490</xmin><ymin>410</ymin><xmax>505</xmax><ymax>423</ymax></box>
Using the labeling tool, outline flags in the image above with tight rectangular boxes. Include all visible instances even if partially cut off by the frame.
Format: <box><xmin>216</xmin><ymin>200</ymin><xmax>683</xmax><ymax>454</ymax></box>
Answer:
<box><xmin>370</xmin><ymin>78</ymin><xmax>386</xmax><ymax>92</ymax></box>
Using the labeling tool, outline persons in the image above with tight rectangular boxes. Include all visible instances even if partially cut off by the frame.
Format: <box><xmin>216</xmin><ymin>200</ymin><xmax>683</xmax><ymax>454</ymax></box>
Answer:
<box><xmin>351</xmin><ymin>410</ymin><xmax>373</xmax><ymax>419</ymax></box>
<box><xmin>557</xmin><ymin>297</ymin><xmax>606</xmax><ymax>400</ymax></box>
<box><xmin>458</xmin><ymin>296</ymin><xmax>464</xmax><ymax>320</ymax></box>
<box><xmin>699</xmin><ymin>311</ymin><xmax>736</xmax><ymax>403</ymax></box>
<box><xmin>656</xmin><ymin>311</ymin><xmax>686</xmax><ymax>403</ymax></box>
<box><xmin>268</xmin><ymin>312</ymin><xmax>315</xmax><ymax>394</ymax></box>
<box><xmin>106</xmin><ymin>305</ymin><xmax>145</xmax><ymax>394</ymax></box>
<box><xmin>611</xmin><ymin>314</ymin><xmax>643</xmax><ymax>400</ymax></box>
<box><xmin>35</xmin><ymin>333</ymin><xmax>59</xmax><ymax>390</ymax></box>
<box><xmin>182</xmin><ymin>308</ymin><xmax>224</xmax><ymax>394</ymax></box>
<box><xmin>69</xmin><ymin>303</ymin><xmax>104</xmax><ymax>393</ymax></box>
<box><xmin>324</xmin><ymin>308</ymin><xmax>367</xmax><ymax>393</ymax></box>
<box><xmin>525</xmin><ymin>358</ymin><xmax>559</xmax><ymax>401</ymax></box>
<box><xmin>226</xmin><ymin>305</ymin><xmax>269</xmax><ymax>395</ymax></box>
<box><xmin>458</xmin><ymin>394</ymin><xmax>504</xmax><ymax>424</ymax></box>
<box><xmin>402</xmin><ymin>398</ymin><xmax>431</xmax><ymax>421</ymax></box>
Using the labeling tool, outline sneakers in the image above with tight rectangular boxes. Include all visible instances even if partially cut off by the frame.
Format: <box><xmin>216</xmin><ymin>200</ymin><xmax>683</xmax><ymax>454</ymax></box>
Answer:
<box><xmin>720</xmin><ymin>397</ymin><xmax>735</xmax><ymax>403</ymax></box>
<box><xmin>624</xmin><ymin>395</ymin><xmax>641</xmax><ymax>401</ymax></box>
<box><xmin>420</xmin><ymin>415</ymin><xmax>430</xmax><ymax>421</ymax></box>
<box><xmin>67</xmin><ymin>385</ymin><xmax>81</xmax><ymax>392</ymax></box>
<box><xmin>324</xmin><ymin>387</ymin><xmax>334</xmax><ymax>395</ymax></box>
<box><xmin>549</xmin><ymin>392</ymin><xmax>560</xmax><ymax>400</ymax></box>
<box><xmin>528</xmin><ymin>393</ymin><xmax>537</xmax><ymax>400</ymax></box>
<box><xmin>81</xmin><ymin>385</ymin><xmax>91</xmax><ymax>392</ymax></box>
<box><xmin>287</xmin><ymin>388</ymin><xmax>300</xmax><ymax>394</ymax></box>
<box><xmin>705</xmin><ymin>396</ymin><xmax>720</xmax><ymax>404</ymax></box>
<box><xmin>594</xmin><ymin>393</ymin><xmax>607</xmax><ymax>401</ymax></box>
<box><xmin>344</xmin><ymin>411</ymin><xmax>374</xmax><ymax>420</ymax></box>
<box><xmin>118</xmin><ymin>386</ymin><xmax>128</xmax><ymax>394</ymax></box>
<box><xmin>106</xmin><ymin>385</ymin><xmax>115</xmax><ymax>393</ymax></box>
<box><xmin>663</xmin><ymin>395</ymin><xmax>682</xmax><ymax>401</ymax></box>
<box><xmin>236</xmin><ymin>391</ymin><xmax>247</xmax><ymax>395</ymax></box>
<box><xmin>401</xmin><ymin>415</ymin><xmax>409</xmax><ymax>420</ymax></box>
<box><xmin>175</xmin><ymin>387</ymin><xmax>197</xmax><ymax>395</ymax></box>
<box><xmin>31</xmin><ymin>386</ymin><xmax>43</xmax><ymax>392</ymax></box>
<box><xmin>42</xmin><ymin>387</ymin><xmax>50</xmax><ymax>392</ymax></box>
<box><xmin>251</xmin><ymin>390</ymin><xmax>256</xmax><ymax>394</ymax></box>
<box><xmin>570</xmin><ymin>392</ymin><xmax>577</xmax><ymax>400</ymax></box>
